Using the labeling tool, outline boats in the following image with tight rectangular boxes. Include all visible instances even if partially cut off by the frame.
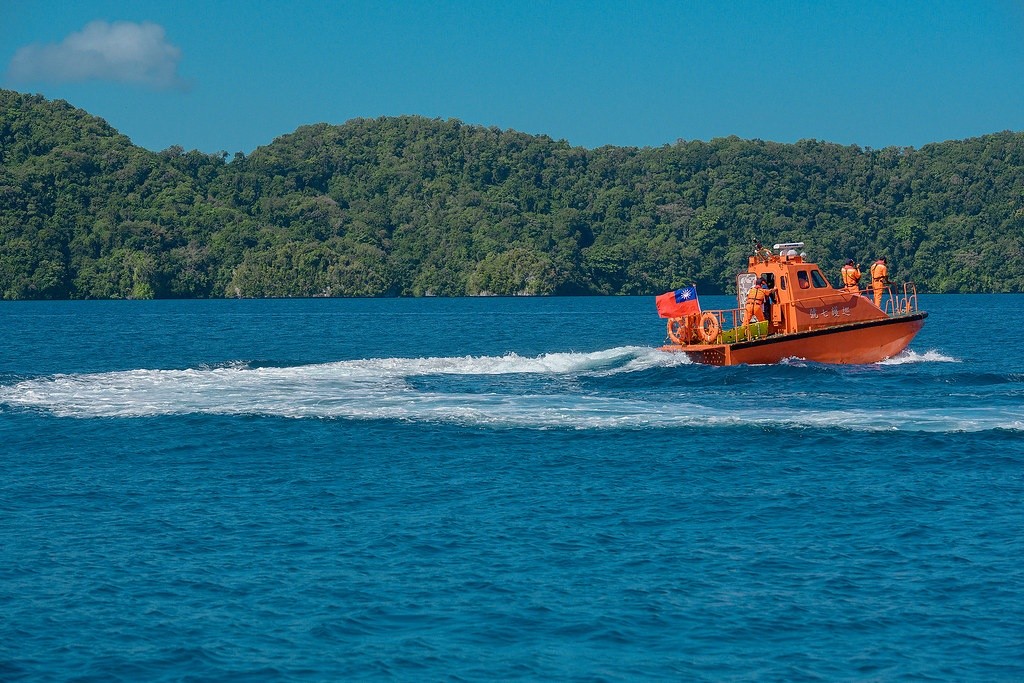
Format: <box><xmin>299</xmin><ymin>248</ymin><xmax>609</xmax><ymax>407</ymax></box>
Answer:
<box><xmin>654</xmin><ymin>241</ymin><xmax>930</xmax><ymax>367</ymax></box>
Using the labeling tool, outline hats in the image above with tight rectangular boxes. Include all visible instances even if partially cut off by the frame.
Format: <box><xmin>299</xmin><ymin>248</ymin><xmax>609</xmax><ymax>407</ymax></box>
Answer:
<box><xmin>880</xmin><ymin>256</ymin><xmax>887</xmax><ymax>264</ymax></box>
<box><xmin>846</xmin><ymin>259</ymin><xmax>855</xmax><ymax>264</ymax></box>
<box><xmin>756</xmin><ymin>279</ymin><xmax>764</xmax><ymax>285</ymax></box>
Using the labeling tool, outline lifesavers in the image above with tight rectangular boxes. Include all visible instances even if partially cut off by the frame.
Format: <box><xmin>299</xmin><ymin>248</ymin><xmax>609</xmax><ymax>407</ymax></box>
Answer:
<box><xmin>665</xmin><ymin>315</ymin><xmax>686</xmax><ymax>346</ymax></box>
<box><xmin>700</xmin><ymin>314</ymin><xmax>720</xmax><ymax>341</ymax></box>
<box><xmin>686</xmin><ymin>311</ymin><xmax>701</xmax><ymax>342</ymax></box>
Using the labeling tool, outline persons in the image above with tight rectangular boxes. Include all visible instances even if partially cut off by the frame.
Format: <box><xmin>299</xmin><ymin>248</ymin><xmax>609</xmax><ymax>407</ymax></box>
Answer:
<box><xmin>870</xmin><ymin>255</ymin><xmax>896</xmax><ymax>309</ymax></box>
<box><xmin>742</xmin><ymin>277</ymin><xmax>778</xmax><ymax>340</ymax></box>
<box><xmin>841</xmin><ymin>259</ymin><xmax>861</xmax><ymax>291</ymax></box>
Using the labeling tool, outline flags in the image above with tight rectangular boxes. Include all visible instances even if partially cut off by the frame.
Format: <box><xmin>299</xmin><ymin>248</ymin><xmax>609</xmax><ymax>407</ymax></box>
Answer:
<box><xmin>655</xmin><ymin>286</ymin><xmax>701</xmax><ymax>319</ymax></box>
